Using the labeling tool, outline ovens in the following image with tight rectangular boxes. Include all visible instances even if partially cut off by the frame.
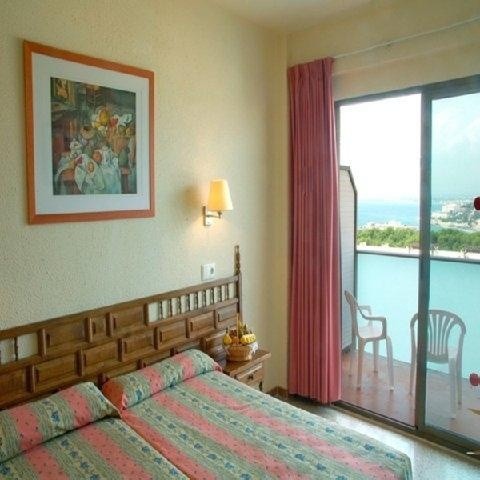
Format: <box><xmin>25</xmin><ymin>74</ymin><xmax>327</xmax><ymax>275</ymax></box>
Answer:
<box><xmin>0</xmin><ymin>244</ymin><xmax>413</xmax><ymax>480</ymax></box>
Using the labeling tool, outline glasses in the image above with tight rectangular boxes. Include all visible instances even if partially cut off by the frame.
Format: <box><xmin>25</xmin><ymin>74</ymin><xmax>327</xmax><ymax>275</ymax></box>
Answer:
<box><xmin>202</xmin><ymin>176</ymin><xmax>236</xmax><ymax>230</ymax></box>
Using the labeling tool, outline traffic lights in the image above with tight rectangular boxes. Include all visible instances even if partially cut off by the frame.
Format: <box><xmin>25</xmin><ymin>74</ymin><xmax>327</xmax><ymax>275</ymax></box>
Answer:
<box><xmin>0</xmin><ymin>379</ymin><xmax>115</xmax><ymax>461</ymax></box>
<box><xmin>103</xmin><ymin>349</ymin><xmax>225</xmax><ymax>411</ymax></box>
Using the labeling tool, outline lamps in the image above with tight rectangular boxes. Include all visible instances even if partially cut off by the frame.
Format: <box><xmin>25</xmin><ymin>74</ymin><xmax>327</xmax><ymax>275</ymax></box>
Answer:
<box><xmin>344</xmin><ymin>288</ymin><xmax>396</xmax><ymax>394</ymax></box>
<box><xmin>407</xmin><ymin>309</ymin><xmax>467</xmax><ymax>419</ymax></box>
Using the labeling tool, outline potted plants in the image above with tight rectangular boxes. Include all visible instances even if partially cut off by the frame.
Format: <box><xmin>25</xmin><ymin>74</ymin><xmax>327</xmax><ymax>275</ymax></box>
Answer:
<box><xmin>22</xmin><ymin>37</ymin><xmax>158</xmax><ymax>227</ymax></box>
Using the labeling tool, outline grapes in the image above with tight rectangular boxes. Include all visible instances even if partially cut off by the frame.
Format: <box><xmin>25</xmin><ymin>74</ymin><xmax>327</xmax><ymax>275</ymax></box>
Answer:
<box><xmin>216</xmin><ymin>351</ymin><xmax>270</xmax><ymax>393</ymax></box>
